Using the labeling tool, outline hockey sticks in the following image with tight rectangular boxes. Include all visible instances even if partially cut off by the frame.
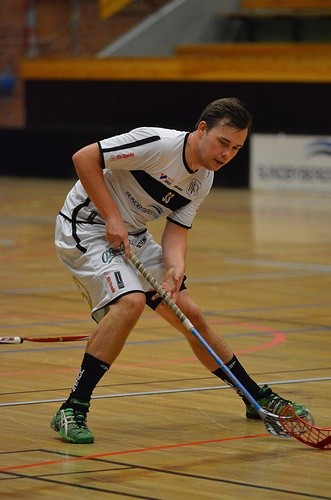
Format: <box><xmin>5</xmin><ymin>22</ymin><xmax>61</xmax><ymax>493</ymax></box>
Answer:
<box><xmin>122</xmin><ymin>244</ymin><xmax>330</xmax><ymax>452</ymax></box>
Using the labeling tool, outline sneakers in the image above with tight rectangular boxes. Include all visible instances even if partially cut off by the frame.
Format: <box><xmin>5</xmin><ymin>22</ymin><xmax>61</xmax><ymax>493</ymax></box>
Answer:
<box><xmin>242</xmin><ymin>384</ymin><xmax>309</xmax><ymax>421</ymax></box>
<box><xmin>50</xmin><ymin>399</ymin><xmax>94</xmax><ymax>444</ymax></box>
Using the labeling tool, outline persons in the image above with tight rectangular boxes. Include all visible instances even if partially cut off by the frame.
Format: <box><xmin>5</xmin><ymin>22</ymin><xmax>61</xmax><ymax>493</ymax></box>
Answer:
<box><xmin>50</xmin><ymin>97</ymin><xmax>311</xmax><ymax>444</ymax></box>
